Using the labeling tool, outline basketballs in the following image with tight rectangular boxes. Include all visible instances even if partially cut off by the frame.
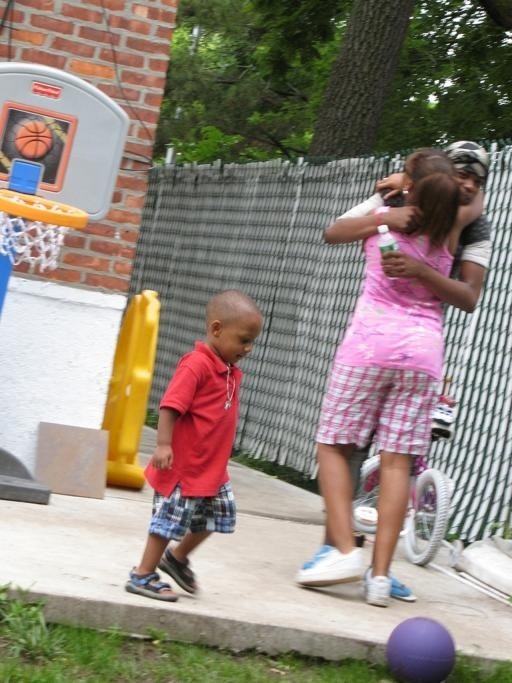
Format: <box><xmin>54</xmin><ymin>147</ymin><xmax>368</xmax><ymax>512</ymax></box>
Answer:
<box><xmin>16</xmin><ymin>121</ymin><xmax>53</xmax><ymax>160</ymax></box>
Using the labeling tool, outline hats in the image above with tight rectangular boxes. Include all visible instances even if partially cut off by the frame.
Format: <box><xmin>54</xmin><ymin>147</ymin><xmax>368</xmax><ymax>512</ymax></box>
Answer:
<box><xmin>443</xmin><ymin>141</ymin><xmax>489</xmax><ymax>185</ymax></box>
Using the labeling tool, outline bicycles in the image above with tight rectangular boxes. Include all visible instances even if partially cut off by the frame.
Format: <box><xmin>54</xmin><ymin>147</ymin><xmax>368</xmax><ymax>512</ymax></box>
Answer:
<box><xmin>351</xmin><ymin>395</ymin><xmax>458</xmax><ymax>566</ymax></box>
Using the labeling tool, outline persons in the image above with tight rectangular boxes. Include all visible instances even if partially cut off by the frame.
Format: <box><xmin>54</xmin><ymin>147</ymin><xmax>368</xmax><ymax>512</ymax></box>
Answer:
<box><xmin>124</xmin><ymin>288</ymin><xmax>266</xmax><ymax>603</ymax></box>
<box><xmin>290</xmin><ymin>146</ymin><xmax>488</xmax><ymax>610</ymax></box>
<box><xmin>316</xmin><ymin>138</ymin><xmax>494</xmax><ymax>604</ymax></box>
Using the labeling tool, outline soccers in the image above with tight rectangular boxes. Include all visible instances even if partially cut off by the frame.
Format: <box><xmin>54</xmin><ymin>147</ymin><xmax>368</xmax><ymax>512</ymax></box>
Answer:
<box><xmin>386</xmin><ymin>616</ymin><xmax>455</xmax><ymax>682</ymax></box>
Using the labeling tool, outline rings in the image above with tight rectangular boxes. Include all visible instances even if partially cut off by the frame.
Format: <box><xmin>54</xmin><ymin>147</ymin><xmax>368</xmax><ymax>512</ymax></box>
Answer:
<box><xmin>381</xmin><ymin>176</ymin><xmax>388</xmax><ymax>182</ymax></box>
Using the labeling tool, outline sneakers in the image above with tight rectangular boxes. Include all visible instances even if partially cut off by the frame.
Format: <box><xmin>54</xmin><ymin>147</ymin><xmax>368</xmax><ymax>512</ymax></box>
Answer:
<box><xmin>293</xmin><ymin>544</ymin><xmax>366</xmax><ymax>587</ymax></box>
<box><xmin>364</xmin><ymin>568</ymin><xmax>393</xmax><ymax>608</ymax></box>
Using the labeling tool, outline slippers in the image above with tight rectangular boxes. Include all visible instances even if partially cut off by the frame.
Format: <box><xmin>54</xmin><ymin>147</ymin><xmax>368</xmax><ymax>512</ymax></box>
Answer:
<box><xmin>387</xmin><ymin>575</ymin><xmax>418</xmax><ymax>602</ymax></box>
<box><xmin>301</xmin><ymin>544</ymin><xmax>336</xmax><ymax>571</ymax></box>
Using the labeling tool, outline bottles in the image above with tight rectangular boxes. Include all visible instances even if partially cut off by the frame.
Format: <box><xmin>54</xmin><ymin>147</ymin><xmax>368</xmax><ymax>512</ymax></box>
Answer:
<box><xmin>375</xmin><ymin>224</ymin><xmax>402</xmax><ymax>284</ymax></box>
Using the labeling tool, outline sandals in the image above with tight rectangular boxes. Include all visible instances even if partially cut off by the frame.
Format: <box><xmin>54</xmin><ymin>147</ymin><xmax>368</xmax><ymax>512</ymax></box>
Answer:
<box><xmin>157</xmin><ymin>548</ymin><xmax>201</xmax><ymax>596</ymax></box>
<box><xmin>124</xmin><ymin>566</ymin><xmax>179</xmax><ymax>602</ymax></box>
